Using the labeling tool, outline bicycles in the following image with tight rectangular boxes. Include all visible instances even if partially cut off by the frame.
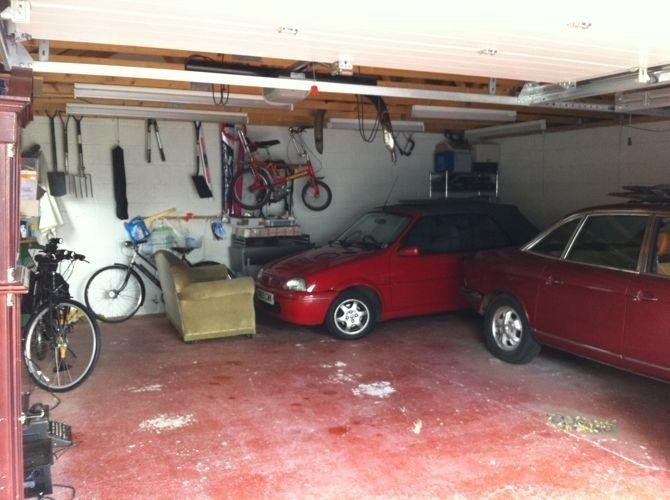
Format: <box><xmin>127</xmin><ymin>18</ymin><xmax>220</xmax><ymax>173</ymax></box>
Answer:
<box><xmin>229</xmin><ymin>122</ymin><xmax>332</xmax><ymax>212</ymax></box>
<box><xmin>84</xmin><ymin>241</ymin><xmax>234</xmax><ymax>326</ymax></box>
<box><xmin>24</xmin><ymin>234</ymin><xmax>104</xmax><ymax>395</ymax></box>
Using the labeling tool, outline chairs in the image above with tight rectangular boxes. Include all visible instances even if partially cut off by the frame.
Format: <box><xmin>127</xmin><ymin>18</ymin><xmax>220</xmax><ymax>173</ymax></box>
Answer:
<box><xmin>153</xmin><ymin>249</ymin><xmax>256</xmax><ymax>345</ymax></box>
<box><xmin>654</xmin><ymin>231</ymin><xmax>670</xmax><ymax>277</ymax></box>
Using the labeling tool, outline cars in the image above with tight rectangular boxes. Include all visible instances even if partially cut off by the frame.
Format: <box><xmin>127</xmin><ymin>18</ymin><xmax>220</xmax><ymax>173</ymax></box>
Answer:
<box><xmin>455</xmin><ymin>183</ymin><xmax>669</xmax><ymax>388</ymax></box>
<box><xmin>252</xmin><ymin>193</ymin><xmax>540</xmax><ymax>344</ymax></box>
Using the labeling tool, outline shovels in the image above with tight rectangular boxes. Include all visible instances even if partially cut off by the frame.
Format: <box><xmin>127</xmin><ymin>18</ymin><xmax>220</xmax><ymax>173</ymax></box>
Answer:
<box><xmin>191</xmin><ymin>121</ymin><xmax>213</xmax><ymax>198</ymax></box>
<box><xmin>44</xmin><ymin>109</ymin><xmax>66</xmax><ymax>196</ymax></box>
